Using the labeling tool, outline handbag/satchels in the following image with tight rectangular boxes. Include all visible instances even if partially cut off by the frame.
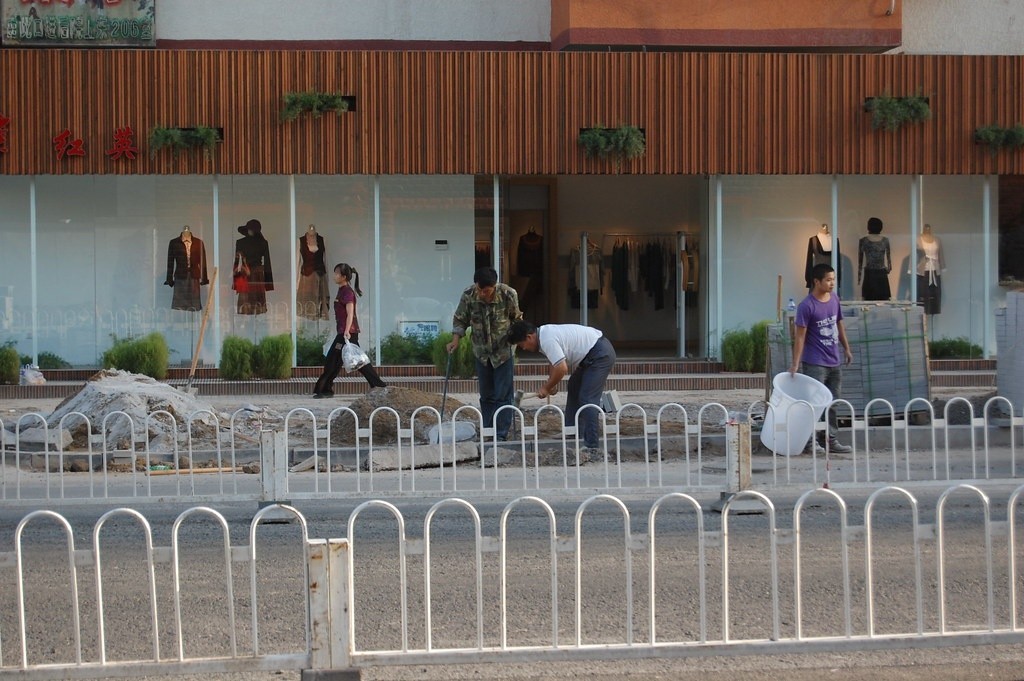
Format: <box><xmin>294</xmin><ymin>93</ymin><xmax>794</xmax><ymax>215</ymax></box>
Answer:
<box><xmin>341</xmin><ymin>338</ymin><xmax>370</xmax><ymax>373</ymax></box>
<box><xmin>20</xmin><ymin>363</ymin><xmax>46</xmax><ymax>385</ymax></box>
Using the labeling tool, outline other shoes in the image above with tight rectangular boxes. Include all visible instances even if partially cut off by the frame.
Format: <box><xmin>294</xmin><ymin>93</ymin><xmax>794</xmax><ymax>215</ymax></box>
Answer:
<box><xmin>313</xmin><ymin>391</ymin><xmax>335</xmax><ymax>399</ymax></box>
<box><xmin>552</xmin><ymin>431</ymin><xmax>575</xmax><ymax>439</ymax></box>
<box><xmin>497</xmin><ymin>436</ymin><xmax>508</xmax><ymax>441</ymax></box>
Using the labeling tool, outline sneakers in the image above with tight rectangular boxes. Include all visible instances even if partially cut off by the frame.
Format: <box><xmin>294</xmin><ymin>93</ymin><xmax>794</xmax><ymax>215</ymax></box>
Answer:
<box><xmin>803</xmin><ymin>440</ymin><xmax>825</xmax><ymax>455</ymax></box>
<box><xmin>823</xmin><ymin>439</ymin><xmax>851</xmax><ymax>452</ymax></box>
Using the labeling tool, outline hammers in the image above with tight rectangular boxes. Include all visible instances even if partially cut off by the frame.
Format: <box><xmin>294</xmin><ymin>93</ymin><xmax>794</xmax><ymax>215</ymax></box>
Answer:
<box><xmin>512</xmin><ymin>388</ymin><xmax>552</xmax><ymax>408</ymax></box>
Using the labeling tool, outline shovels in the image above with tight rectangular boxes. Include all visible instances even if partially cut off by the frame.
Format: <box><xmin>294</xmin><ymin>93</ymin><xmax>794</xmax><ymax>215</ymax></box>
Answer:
<box><xmin>199</xmin><ymin>416</ymin><xmax>262</xmax><ymax>445</ymax></box>
<box><xmin>144</xmin><ymin>453</ymin><xmax>328</xmax><ymax>475</ymax></box>
<box><xmin>173</xmin><ymin>265</ymin><xmax>221</xmax><ymax>401</ymax></box>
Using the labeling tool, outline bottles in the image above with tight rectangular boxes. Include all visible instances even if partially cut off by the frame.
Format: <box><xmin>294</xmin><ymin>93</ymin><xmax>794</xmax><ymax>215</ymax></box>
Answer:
<box><xmin>787</xmin><ymin>299</ymin><xmax>795</xmax><ymax>315</ymax></box>
<box><xmin>20</xmin><ymin>364</ymin><xmax>26</xmax><ymax>386</ymax></box>
<box><xmin>149</xmin><ymin>465</ymin><xmax>169</xmax><ymax>471</ymax></box>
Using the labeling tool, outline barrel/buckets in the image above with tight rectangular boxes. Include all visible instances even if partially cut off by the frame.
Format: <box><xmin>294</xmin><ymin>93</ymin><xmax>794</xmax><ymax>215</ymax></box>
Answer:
<box><xmin>760</xmin><ymin>372</ymin><xmax>833</xmax><ymax>455</ymax></box>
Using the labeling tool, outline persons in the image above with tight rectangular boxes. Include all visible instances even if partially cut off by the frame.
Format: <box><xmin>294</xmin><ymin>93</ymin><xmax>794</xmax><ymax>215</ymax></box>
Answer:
<box><xmin>445</xmin><ymin>266</ymin><xmax>524</xmax><ymax>442</ymax></box>
<box><xmin>805</xmin><ymin>224</ymin><xmax>843</xmax><ymax>301</ymax></box>
<box><xmin>787</xmin><ymin>263</ymin><xmax>854</xmax><ymax>456</ymax></box>
<box><xmin>505</xmin><ymin>320</ymin><xmax>616</xmax><ymax>458</ymax></box>
<box><xmin>162</xmin><ymin>224</ymin><xmax>209</xmax><ymax>312</ymax></box>
<box><xmin>295</xmin><ymin>224</ymin><xmax>330</xmax><ymax>321</ymax></box>
<box><xmin>906</xmin><ymin>221</ymin><xmax>944</xmax><ymax>315</ymax></box>
<box><xmin>856</xmin><ymin>215</ymin><xmax>892</xmax><ymax>302</ymax></box>
<box><xmin>312</xmin><ymin>263</ymin><xmax>386</xmax><ymax>399</ymax></box>
<box><xmin>230</xmin><ymin>219</ymin><xmax>274</xmax><ymax>316</ymax></box>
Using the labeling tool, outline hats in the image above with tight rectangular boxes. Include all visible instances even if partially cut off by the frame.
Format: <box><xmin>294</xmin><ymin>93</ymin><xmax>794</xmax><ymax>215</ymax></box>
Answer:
<box><xmin>238</xmin><ymin>219</ymin><xmax>262</xmax><ymax>237</ymax></box>
